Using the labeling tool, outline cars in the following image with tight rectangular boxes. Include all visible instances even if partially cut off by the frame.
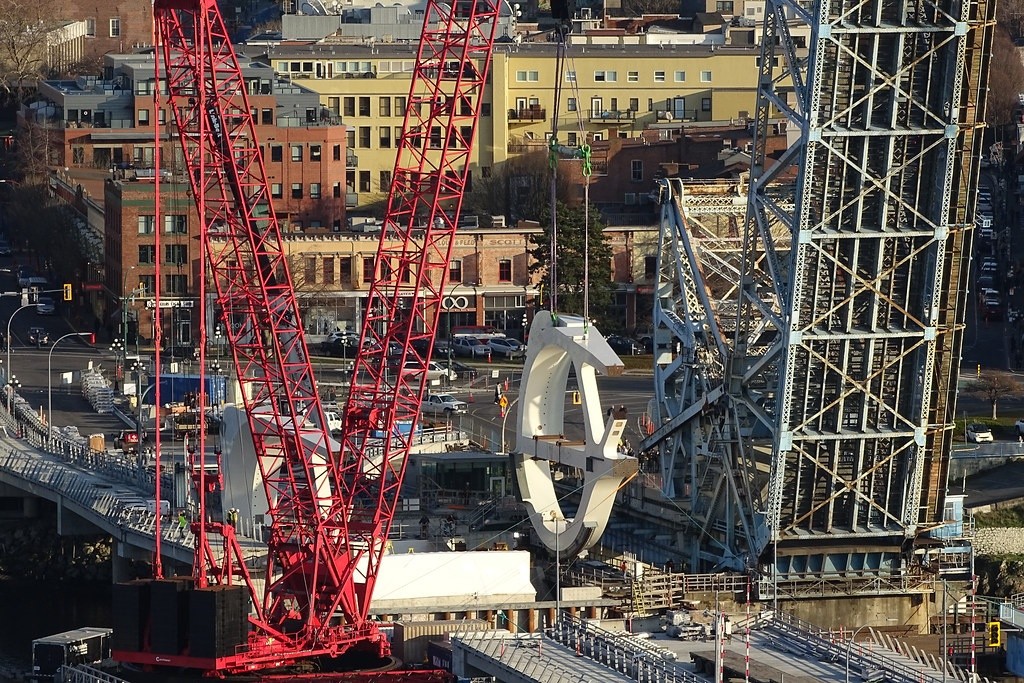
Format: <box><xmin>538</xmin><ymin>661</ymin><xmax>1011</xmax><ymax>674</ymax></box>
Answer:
<box><xmin>328</xmin><ymin>332</ymin><xmax>377</xmax><ymax>348</ymax></box>
<box><xmin>111</xmin><ymin>429</ymin><xmax>152</xmax><ymax>455</ymax></box>
<box><xmin>967</xmin><ymin>418</ymin><xmax>1024</xmax><ymax>443</ymax></box>
<box><xmin>150</xmin><ymin>346</ymin><xmax>196</xmax><ymax>365</ymax></box>
<box><xmin>607</xmin><ymin>325</ymin><xmax>655</xmax><ymax>355</ymax></box>
<box><xmin>370</xmin><ymin>356</ymin><xmax>479</xmax><ymax>383</ymax></box>
<box><xmin>390</xmin><ymin>341</ymin><xmax>451</xmax><ymax>357</ymax></box>
<box><xmin>16</xmin><ymin>264</ymin><xmax>57</xmax><ymax>350</ymax></box>
<box><xmin>491</xmin><ymin>337</ymin><xmax>522</xmax><ymax>355</ymax></box>
<box><xmin>324</xmin><ymin>412</ymin><xmax>343</xmax><ymax>431</ymax></box>
<box><xmin>977</xmin><ymin>229</ymin><xmax>1002</xmax><ymax>318</ymax></box>
<box><xmin>27</xmin><ymin>327</ymin><xmax>50</xmax><ymax>346</ymax></box>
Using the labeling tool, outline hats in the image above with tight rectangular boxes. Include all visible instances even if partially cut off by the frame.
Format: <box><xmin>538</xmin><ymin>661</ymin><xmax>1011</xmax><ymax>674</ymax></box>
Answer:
<box><xmin>497</xmin><ymin>382</ymin><xmax>499</xmax><ymax>384</ymax></box>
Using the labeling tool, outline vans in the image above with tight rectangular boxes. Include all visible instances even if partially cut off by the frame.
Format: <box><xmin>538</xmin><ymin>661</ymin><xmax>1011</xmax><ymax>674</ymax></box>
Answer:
<box><xmin>450</xmin><ymin>325</ymin><xmax>506</xmax><ymax>343</ymax></box>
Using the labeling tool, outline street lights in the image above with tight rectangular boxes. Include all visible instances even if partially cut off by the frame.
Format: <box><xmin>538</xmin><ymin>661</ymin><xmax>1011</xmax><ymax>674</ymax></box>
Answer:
<box><xmin>341</xmin><ymin>331</ymin><xmax>348</xmax><ymax>397</ymax></box>
<box><xmin>7</xmin><ymin>303</ymin><xmax>47</xmax><ymax>418</ymax></box>
<box><xmin>108</xmin><ymin>338</ymin><xmax>124</xmax><ymax>394</ymax></box>
<box><xmin>447</xmin><ymin>281</ymin><xmax>472</xmax><ymax>386</ymax></box>
<box><xmin>549</xmin><ymin>511</ymin><xmax>899</xmax><ymax>683</ymax></box>
<box><xmin>522</xmin><ymin>314</ymin><xmax>528</xmax><ymax>364</ymax></box>
<box><xmin>50</xmin><ymin>333</ymin><xmax>94</xmax><ymax>443</ymax></box>
<box><xmin>138</xmin><ymin>380</ymin><xmax>167</xmax><ymax>472</ymax></box>
<box><xmin>6</xmin><ymin>375</ymin><xmax>22</xmax><ymax>429</ymax></box>
<box><xmin>108</xmin><ymin>266</ymin><xmax>146</xmax><ymax>470</ymax></box>
<box><xmin>210</xmin><ymin>326</ymin><xmax>221</xmax><ymax>418</ymax></box>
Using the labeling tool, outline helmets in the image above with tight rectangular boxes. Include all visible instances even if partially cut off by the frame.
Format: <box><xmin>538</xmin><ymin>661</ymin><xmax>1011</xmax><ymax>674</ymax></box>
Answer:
<box><xmin>236</xmin><ymin>509</ymin><xmax>239</xmax><ymax>513</ymax></box>
<box><xmin>230</xmin><ymin>508</ymin><xmax>234</xmax><ymax>511</ymax></box>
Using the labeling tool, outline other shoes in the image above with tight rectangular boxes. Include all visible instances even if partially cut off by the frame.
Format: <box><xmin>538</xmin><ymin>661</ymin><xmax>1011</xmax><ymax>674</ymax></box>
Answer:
<box><xmin>494</xmin><ymin>402</ymin><xmax>498</xmax><ymax>404</ymax></box>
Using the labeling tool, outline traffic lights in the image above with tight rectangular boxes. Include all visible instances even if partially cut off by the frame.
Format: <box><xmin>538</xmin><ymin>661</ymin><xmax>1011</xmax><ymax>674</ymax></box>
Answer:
<box><xmin>989</xmin><ymin>622</ymin><xmax>1001</xmax><ymax>648</ymax></box>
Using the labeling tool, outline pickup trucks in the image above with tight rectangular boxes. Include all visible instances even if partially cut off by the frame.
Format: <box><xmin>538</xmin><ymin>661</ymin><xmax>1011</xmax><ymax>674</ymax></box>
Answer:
<box><xmin>405</xmin><ymin>394</ymin><xmax>469</xmax><ymax>417</ymax></box>
<box><xmin>320</xmin><ymin>338</ymin><xmax>357</xmax><ymax>358</ymax></box>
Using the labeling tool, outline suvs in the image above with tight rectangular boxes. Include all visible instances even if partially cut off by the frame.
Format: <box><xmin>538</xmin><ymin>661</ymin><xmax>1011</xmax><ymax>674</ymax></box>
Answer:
<box><xmin>452</xmin><ymin>336</ymin><xmax>492</xmax><ymax>358</ymax></box>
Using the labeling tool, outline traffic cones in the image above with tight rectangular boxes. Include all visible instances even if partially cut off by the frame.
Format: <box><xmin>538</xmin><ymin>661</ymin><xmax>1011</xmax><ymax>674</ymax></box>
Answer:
<box><xmin>486</xmin><ymin>352</ymin><xmax>508</xmax><ymax>391</ymax></box>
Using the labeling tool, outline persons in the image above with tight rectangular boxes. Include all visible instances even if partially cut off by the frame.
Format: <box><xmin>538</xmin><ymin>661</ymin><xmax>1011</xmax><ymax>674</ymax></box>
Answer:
<box><xmin>617</xmin><ymin>438</ymin><xmax>660</xmax><ymax>466</ymax></box>
<box><xmin>494</xmin><ymin>382</ymin><xmax>508</xmax><ymax>417</ymax></box>
<box><xmin>226</xmin><ymin>508</ymin><xmax>240</xmax><ymax>529</ymax></box>
<box><xmin>619</xmin><ymin>405</ymin><xmax>627</xmax><ymax>414</ymax></box>
<box><xmin>607</xmin><ymin>405</ymin><xmax>615</xmax><ymax>416</ymax></box>
<box><xmin>622</xmin><ymin>562</ymin><xmax>627</xmax><ymax>583</ymax></box>
<box><xmin>178</xmin><ymin>510</ymin><xmax>188</xmax><ymax>537</ymax></box>
<box><xmin>419</xmin><ymin>515</ymin><xmax>430</xmax><ymax>525</ymax></box>
